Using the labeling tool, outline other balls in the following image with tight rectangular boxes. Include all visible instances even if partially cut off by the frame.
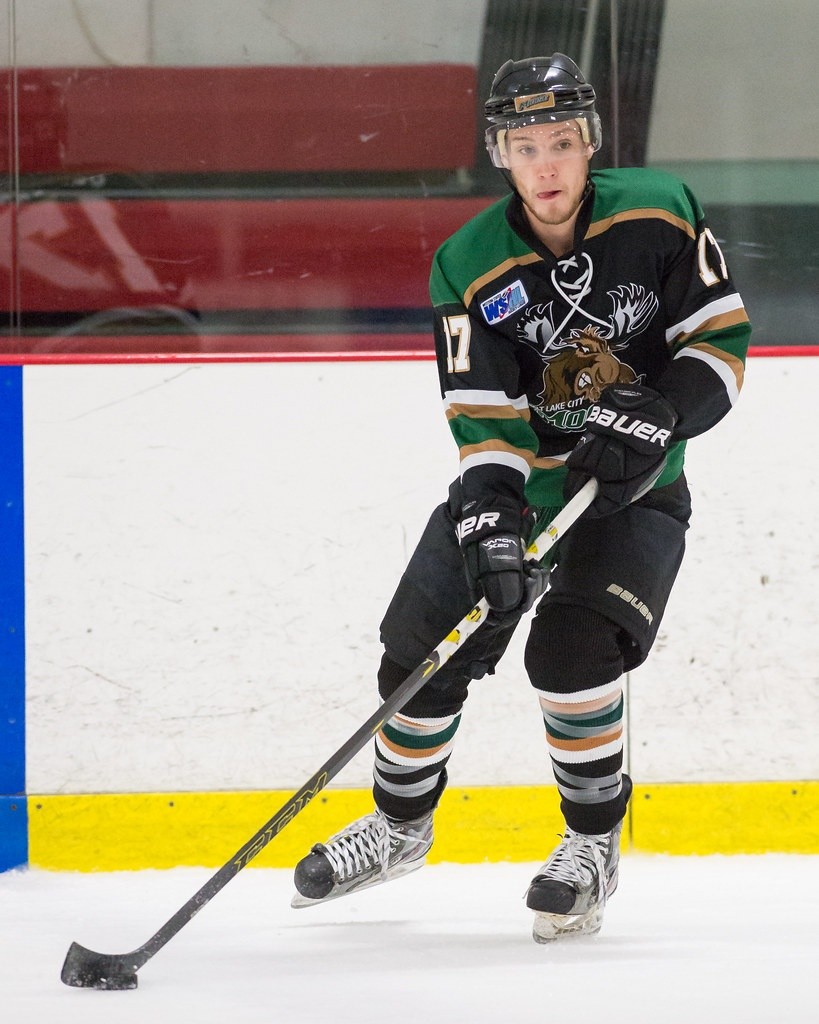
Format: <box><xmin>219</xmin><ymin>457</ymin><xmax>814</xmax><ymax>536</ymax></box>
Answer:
<box><xmin>96</xmin><ymin>972</ymin><xmax>140</xmax><ymax>991</ymax></box>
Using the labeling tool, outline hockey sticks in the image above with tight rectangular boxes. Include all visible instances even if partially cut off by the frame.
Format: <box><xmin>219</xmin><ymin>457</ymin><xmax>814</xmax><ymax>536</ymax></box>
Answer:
<box><xmin>58</xmin><ymin>475</ymin><xmax>603</xmax><ymax>994</ymax></box>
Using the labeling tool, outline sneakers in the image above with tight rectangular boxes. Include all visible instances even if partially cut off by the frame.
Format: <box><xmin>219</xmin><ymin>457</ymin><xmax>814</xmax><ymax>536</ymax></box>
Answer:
<box><xmin>290</xmin><ymin>808</ymin><xmax>434</xmax><ymax>909</ymax></box>
<box><xmin>523</xmin><ymin>818</ymin><xmax>624</xmax><ymax>944</ymax></box>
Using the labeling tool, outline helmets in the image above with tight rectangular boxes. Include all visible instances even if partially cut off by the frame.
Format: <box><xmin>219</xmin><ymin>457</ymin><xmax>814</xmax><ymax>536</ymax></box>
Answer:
<box><xmin>483</xmin><ymin>53</ymin><xmax>597</xmax><ymax>148</ymax></box>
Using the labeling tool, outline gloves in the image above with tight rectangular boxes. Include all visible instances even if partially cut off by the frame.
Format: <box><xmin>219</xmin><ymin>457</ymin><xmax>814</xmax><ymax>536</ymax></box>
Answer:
<box><xmin>562</xmin><ymin>382</ymin><xmax>679</xmax><ymax>520</ymax></box>
<box><xmin>445</xmin><ymin>495</ymin><xmax>550</xmax><ymax>626</ymax></box>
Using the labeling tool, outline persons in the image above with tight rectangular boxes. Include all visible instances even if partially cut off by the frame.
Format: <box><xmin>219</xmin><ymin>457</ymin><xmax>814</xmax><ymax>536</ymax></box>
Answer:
<box><xmin>291</xmin><ymin>52</ymin><xmax>754</xmax><ymax>944</ymax></box>
